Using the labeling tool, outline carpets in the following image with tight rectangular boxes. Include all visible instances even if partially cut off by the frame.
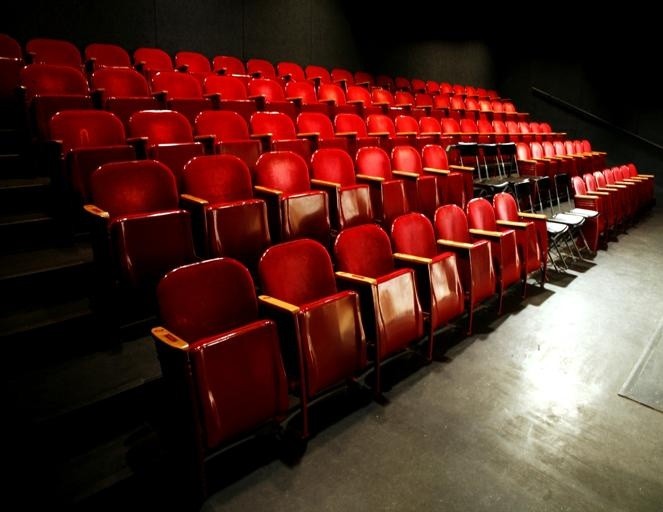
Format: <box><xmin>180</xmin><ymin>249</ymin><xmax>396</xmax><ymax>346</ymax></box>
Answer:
<box><xmin>617</xmin><ymin>316</ymin><xmax>662</xmax><ymax>414</ymax></box>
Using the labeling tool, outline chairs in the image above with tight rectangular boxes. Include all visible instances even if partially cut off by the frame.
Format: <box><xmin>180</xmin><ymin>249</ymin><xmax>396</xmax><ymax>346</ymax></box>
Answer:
<box><xmin>539</xmin><ymin>122</ymin><xmax>567</xmax><ymax>142</ymax></box>
<box><xmin>44</xmin><ymin>109</ymin><xmax>149</xmax><ymax>196</ymax></box>
<box><xmin>514</xmin><ymin>179</ymin><xmax>575</xmax><ymax>281</ymax></box>
<box><xmin>464</xmin><ymin>97</ymin><xmax>493</xmax><ymax>123</ymax></box>
<box><xmin>346</xmin><ymin>86</ymin><xmax>389</xmax><ymax>115</ymax></box>
<box><xmin>376</xmin><ymin>75</ymin><xmax>401</xmax><ymax>91</ymax></box>
<box><xmin>259</xmin><ymin>237</ymin><xmax>376</xmax><ymax>439</ymax></box>
<box><xmin>203</xmin><ymin>75</ymin><xmax>265</xmax><ymax>120</ymax></box>
<box><xmin>334</xmin><ymin>113</ymin><xmax>388</xmax><ymax>155</ymax></box>
<box><xmin>490</xmin><ymin>121</ymin><xmax>519</xmax><ymax>143</ymax></box>
<box><xmin>573</xmin><ymin>140</ymin><xmax>600</xmax><ymax>171</ymax></box>
<box><xmin>460</xmin><ymin>118</ymin><xmax>489</xmax><ymax>143</ymax></box>
<box><xmin>494</xmin><ymin>192</ymin><xmax>549</xmax><ymax>300</ymax></box>
<box><xmin>175</xmin><ymin>52</ymin><xmax>226</xmax><ymax>82</ymax></box>
<box><xmin>331</xmin><ymin>68</ymin><xmax>370</xmax><ymax>92</ymax></box>
<box><xmin>394</xmin><ymin>77</ymin><xmax>425</xmax><ymax>91</ymax></box>
<box><xmin>285</xmin><ymin>80</ymin><xmax>334</xmax><ymax>117</ymax></box>
<box><xmin>628</xmin><ymin>163</ymin><xmax>654</xmax><ymax>200</ymax></box>
<box><xmin>477</xmin><ymin>120</ymin><xmax>505</xmax><ymax>143</ymax></box>
<box><xmin>212</xmin><ymin>55</ymin><xmax>261</xmax><ymax>82</ymax></box>
<box><xmin>0</xmin><ymin>36</ymin><xmax>35</xmax><ymax>96</ymax></box>
<box><xmin>476</xmin><ymin>87</ymin><xmax>491</xmax><ymax>100</ymax></box>
<box><xmin>570</xmin><ymin>176</ymin><xmax>609</xmax><ymax>255</ymax></box>
<box><xmin>491</xmin><ymin>101</ymin><xmax>518</xmax><ymax>122</ymax></box>
<box><xmin>395</xmin><ymin>115</ymin><xmax>440</xmax><ymax>149</ymax></box>
<box><xmin>612</xmin><ymin>166</ymin><xmax>642</xmax><ymax>213</ymax></box>
<box><xmin>517</xmin><ymin>122</ymin><xmax>543</xmax><ymax>142</ymax></box>
<box><xmin>150</xmin><ymin>257</ymin><xmax>300</xmax><ymax>452</ymax></box>
<box><xmin>357</xmin><ymin>145</ymin><xmax>418</xmax><ymax>224</ymax></box>
<box><xmin>554</xmin><ymin>174</ymin><xmax>599</xmax><ymax>260</ymax></box>
<box><xmin>392</xmin><ymin>145</ymin><xmax>451</xmax><ymax>209</ymax></box>
<box><xmin>500</xmin><ymin>142</ymin><xmax>543</xmax><ymax>209</ymax></box>
<box><xmin>478</xmin><ymin>101</ymin><xmax>506</xmax><ymax>123</ymax></box>
<box><xmin>487</xmin><ymin>89</ymin><xmax>511</xmax><ymax>102</ymax></box>
<box><xmin>514</xmin><ymin>143</ymin><xmax>551</xmax><ymax>178</ymax></box>
<box><xmin>459</xmin><ymin>141</ymin><xmax>508</xmax><ymax>204</ymax></box>
<box><xmin>503</xmin><ymin>102</ymin><xmax>530</xmax><ymax>123</ymax></box>
<box><xmin>453</xmin><ymin>83</ymin><xmax>468</xmax><ymax>97</ymax></box>
<box><xmin>318</xmin><ymin>85</ymin><xmax>363</xmax><ymax>117</ymax></box>
<box><xmin>478</xmin><ymin>143</ymin><xmax>534</xmax><ymax>212</ymax></box>
<box><xmin>603</xmin><ymin>168</ymin><xmax>634</xmax><ymax>216</ymax></box>
<box><xmin>367</xmin><ymin>114</ymin><xmax>417</xmax><ymax>152</ymax></box>
<box><xmin>468</xmin><ymin>196</ymin><xmax>528</xmax><ymax>314</ymax></box>
<box><xmin>26</xmin><ymin>39</ymin><xmax>96</xmax><ymax>72</ymax></box>
<box><xmin>193</xmin><ymin>111</ymin><xmax>271</xmax><ymax>172</ymax></box>
<box><xmin>439</xmin><ymin>82</ymin><xmax>454</xmax><ymax>95</ymax></box>
<box><xmin>151</xmin><ymin>71</ymin><xmax>220</xmax><ymax>122</ymax></box>
<box><xmin>529</xmin><ymin>142</ymin><xmax>563</xmax><ymax>177</ymax></box>
<box><xmin>85</xmin><ymin>43</ymin><xmax>145</xmax><ymax>71</ymax></box>
<box><xmin>251</xmin><ymin>110</ymin><xmax>318</xmax><ymax>160</ymax></box>
<box><xmin>529</xmin><ymin>121</ymin><xmax>553</xmax><ymax>143</ymax></box>
<box><xmin>372</xmin><ymin>86</ymin><xmax>413</xmax><ymax>117</ymax></box>
<box><xmin>441</xmin><ymin>118</ymin><xmax>480</xmax><ymax>143</ymax></box>
<box><xmin>354</xmin><ymin>71</ymin><xmax>374</xmax><ymax>90</ymax></box>
<box><xmin>248</xmin><ymin>78</ymin><xmax>303</xmax><ymax>120</ymax></box>
<box><xmin>553</xmin><ymin>141</ymin><xmax>573</xmax><ymax>174</ymax></box>
<box><xmin>466</xmin><ymin>85</ymin><xmax>479</xmax><ymax>99</ymax></box>
<box><xmin>433</xmin><ymin>93</ymin><xmax>465</xmax><ymax>120</ymax></box>
<box><xmin>564</xmin><ymin>141</ymin><xmax>591</xmax><ymax>174</ymax></box>
<box><xmin>18</xmin><ymin>63</ymin><xmax>105</xmax><ymax>140</ymax></box>
<box><xmin>423</xmin><ymin>144</ymin><xmax>474</xmax><ymax>212</ymax></box>
<box><xmin>450</xmin><ymin>96</ymin><xmax>480</xmax><ymax>122</ymax></box>
<box><xmin>90</xmin><ymin>65</ymin><xmax>168</xmax><ymax>131</ymax></box>
<box><xmin>135</xmin><ymin>48</ymin><xmax>188</xmax><ymax>82</ymax></box>
<box><xmin>412</xmin><ymin>79</ymin><xmax>429</xmax><ymax>93</ymax></box>
<box><xmin>391</xmin><ymin>212</ymin><xmax>474</xmax><ymax>361</ymax></box>
<box><xmin>581</xmin><ymin>140</ymin><xmax>607</xmax><ymax>169</ymax></box>
<box><xmin>334</xmin><ymin>224</ymin><xmax>433</xmax><ymax>395</ymax></box>
<box><xmin>180</xmin><ymin>155</ymin><xmax>283</xmax><ymax>256</ymax></box>
<box><xmin>311</xmin><ymin>149</ymin><xmax>384</xmax><ymax>229</ymax></box>
<box><xmin>593</xmin><ymin>172</ymin><xmax>627</xmax><ymax>223</ymax></box>
<box><xmin>305</xmin><ymin>65</ymin><xmax>347</xmax><ymax>86</ymax></box>
<box><xmin>278</xmin><ymin>62</ymin><xmax>322</xmax><ymax>85</ymax></box>
<box><xmin>128</xmin><ymin>109</ymin><xmax>215</xmax><ymax>186</ymax></box>
<box><xmin>246</xmin><ymin>59</ymin><xmax>293</xmax><ymax>81</ymax></box>
<box><xmin>253</xmin><ymin>152</ymin><xmax>340</xmax><ymax>238</ymax></box>
<box><xmin>506</xmin><ymin>121</ymin><xmax>533</xmax><ymax>143</ymax></box>
<box><xmin>434</xmin><ymin>204</ymin><xmax>505</xmax><ymax>335</ymax></box>
<box><xmin>536</xmin><ymin>175</ymin><xmax>586</xmax><ymax>270</ymax></box>
<box><xmin>583</xmin><ymin>172</ymin><xmax>618</xmax><ymax>236</ymax></box>
<box><xmin>297</xmin><ymin>111</ymin><xmax>356</xmax><ymax>153</ymax></box>
<box><xmin>395</xmin><ymin>91</ymin><xmax>432</xmax><ymax>116</ymax></box>
<box><xmin>619</xmin><ymin>165</ymin><xmax>648</xmax><ymax>205</ymax></box>
<box><xmin>415</xmin><ymin>93</ymin><xmax>449</xmax><ymax>119</ymax></box>
<box><xmin>425</xmin><ymin>81</ymin><xmax>443</xmax><ymax>97</ymax></box>
<box><xmin>82</xmin><ymin>160</ymin><xmax>207</xmax><ymax>273</ymax></box>
<box><xmin>420</xmin><ymin>117</ymin><xmax>462</xmax><ymax>149</ymax></box>
